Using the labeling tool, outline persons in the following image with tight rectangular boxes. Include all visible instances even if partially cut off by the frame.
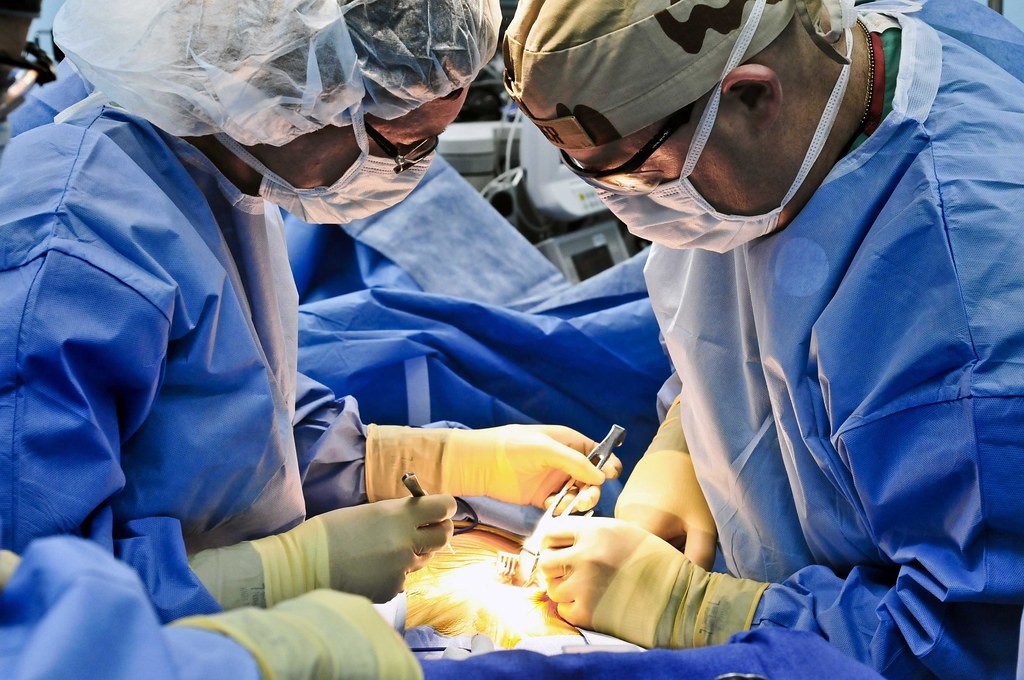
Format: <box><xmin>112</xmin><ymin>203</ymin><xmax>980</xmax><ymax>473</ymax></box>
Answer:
<box><xmin>0</xmin><ymin>0</ymin><xmax>1024</xmax><ymax>680</ymax></box>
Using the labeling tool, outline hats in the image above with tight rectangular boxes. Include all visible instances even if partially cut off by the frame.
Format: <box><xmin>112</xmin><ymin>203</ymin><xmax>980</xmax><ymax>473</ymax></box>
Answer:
<box><xmin>500</xmin><ymin>0</ymin><xmax>796</xmax><ymax>149</ymax></box>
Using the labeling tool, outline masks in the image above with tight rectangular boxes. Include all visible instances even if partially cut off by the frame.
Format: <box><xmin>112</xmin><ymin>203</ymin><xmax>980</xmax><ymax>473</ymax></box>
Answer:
<box><xmin>213</xmin><ymin>112</ymin><xmax>435</xmax><ymax>225</ymax></box>
<box><xmin>596</xmin><ymin>96</ymin><xmax>819</xmax><ymax>253</ymax></box>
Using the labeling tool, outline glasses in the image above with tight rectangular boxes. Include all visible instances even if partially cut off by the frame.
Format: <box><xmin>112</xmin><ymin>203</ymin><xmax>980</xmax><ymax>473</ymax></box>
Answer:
<box><xmin>559</xmin><ymin>102</ymin><xmax>695</xmax><ymax>200</ymax></box>
<box><xmin>0</xmin><ymin>41</ymin><xmax>52</xmax><ymax>116</ymax></box>
<box><xmin>363</xmin><ymin>120</ymin><xmax>438</xmax><ymax>174</ymax></box>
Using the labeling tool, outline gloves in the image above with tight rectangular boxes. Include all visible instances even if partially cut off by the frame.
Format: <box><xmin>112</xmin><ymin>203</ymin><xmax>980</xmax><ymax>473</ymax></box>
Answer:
<box><xmin>541</xmin><ymin>517</ymin><xmax>771</xmax><ymax>652</ymax></box>
<box><xmin>364</xmin><ymin>422</ymin><xmax>623</xmax><ymax>516</ymax></box>
<box><xmin>167</xmin><ymin>588</ymin><xmax>424</xmax><ymax>680</ymax></box>
<box><xmin>614</xmin><ymin>395</ymin><xmax>717</xmax><ymax>572</ymax></box>
<box><xmin>187</xmin><ymin>489</ymin><xmax>457</xmax><ymax>610</ymax></box>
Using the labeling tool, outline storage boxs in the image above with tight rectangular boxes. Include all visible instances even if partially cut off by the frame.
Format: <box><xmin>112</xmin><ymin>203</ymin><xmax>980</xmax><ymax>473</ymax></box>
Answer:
<box><xmin>536</xmin><ymin>220</ymin><xmax>630</xmax><ymax>286</ymax></box>
